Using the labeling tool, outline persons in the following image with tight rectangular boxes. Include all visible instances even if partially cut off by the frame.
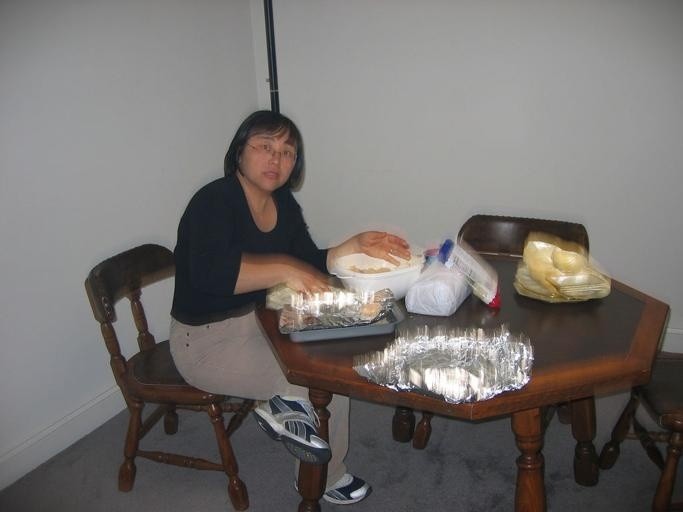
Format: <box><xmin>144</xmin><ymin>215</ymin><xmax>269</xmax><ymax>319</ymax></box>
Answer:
<box><xmin>169</xmin><ymin>111</ymin><xmax>412</xmax><ymax>506</ymax></box>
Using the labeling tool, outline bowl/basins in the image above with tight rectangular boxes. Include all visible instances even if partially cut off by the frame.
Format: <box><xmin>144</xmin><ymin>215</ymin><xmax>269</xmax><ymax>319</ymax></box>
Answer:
<box><xmin>326</xmin><ymin>249</ymin><xmax>427</xmax><ymax>305</ymax></box>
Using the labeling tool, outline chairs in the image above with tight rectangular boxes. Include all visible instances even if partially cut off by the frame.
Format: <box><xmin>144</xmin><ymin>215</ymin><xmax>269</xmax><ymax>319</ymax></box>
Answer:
<box><xmin>598</xmin><ymin>351</ymin><xmax>682</xmax><ymax>512</ymax></box>
<box><xmin>411</xmin><ymin>214</ymin><xmax>592</xmax><ymax>451</ymax></box>
<box><xmin>84</xmin><ymin>242</ymin><xmax>256</xmax><ymax>510</ymax></box>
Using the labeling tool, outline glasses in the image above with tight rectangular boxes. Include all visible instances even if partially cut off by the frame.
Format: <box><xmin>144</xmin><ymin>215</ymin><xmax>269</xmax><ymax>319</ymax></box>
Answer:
<box><xmin>246</xmin><ymin>142</ymin><xmax>298</xmax><ymax>164</ymax></box>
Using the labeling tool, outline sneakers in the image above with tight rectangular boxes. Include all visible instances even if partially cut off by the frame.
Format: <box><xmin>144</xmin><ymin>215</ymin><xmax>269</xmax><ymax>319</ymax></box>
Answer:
<box><xmin>252</xmin><ymin>395</ymin><xmax>331</xmax><ymax>466</ymax></box>
<box><xmin>294</xmin><ymin>472</ymin><xmax>372</xmax><ymax>505</ymax></box>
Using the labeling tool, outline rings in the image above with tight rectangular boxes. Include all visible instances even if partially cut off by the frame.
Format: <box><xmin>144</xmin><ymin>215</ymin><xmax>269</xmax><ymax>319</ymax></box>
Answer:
<box><xmin>387</xmin><ymin>247</ymin><xmax>395</xmax><ymax>256</ymax></box>
